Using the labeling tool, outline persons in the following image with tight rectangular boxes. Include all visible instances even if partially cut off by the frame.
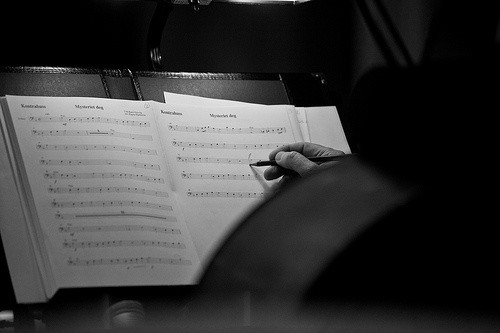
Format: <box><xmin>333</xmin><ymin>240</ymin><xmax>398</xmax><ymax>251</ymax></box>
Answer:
<box><xmin>262</xmin><ymin>0</ymin><xmax>500</xmax><ymax>320</ymax></box>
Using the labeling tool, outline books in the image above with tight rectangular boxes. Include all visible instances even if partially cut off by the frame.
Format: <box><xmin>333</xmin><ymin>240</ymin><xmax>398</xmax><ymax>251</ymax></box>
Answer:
<box><xmin>0</xmin><ymin>94</ymin><xmax>306</xmax><ymax>305</ymax></box>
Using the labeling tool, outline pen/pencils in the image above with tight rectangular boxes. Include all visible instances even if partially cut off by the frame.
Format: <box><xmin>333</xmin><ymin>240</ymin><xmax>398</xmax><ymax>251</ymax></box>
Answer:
<box><xmin>250</xmin><ymin>153</ymin><xmax>353</xmax><ymax>166</ymax></box>
